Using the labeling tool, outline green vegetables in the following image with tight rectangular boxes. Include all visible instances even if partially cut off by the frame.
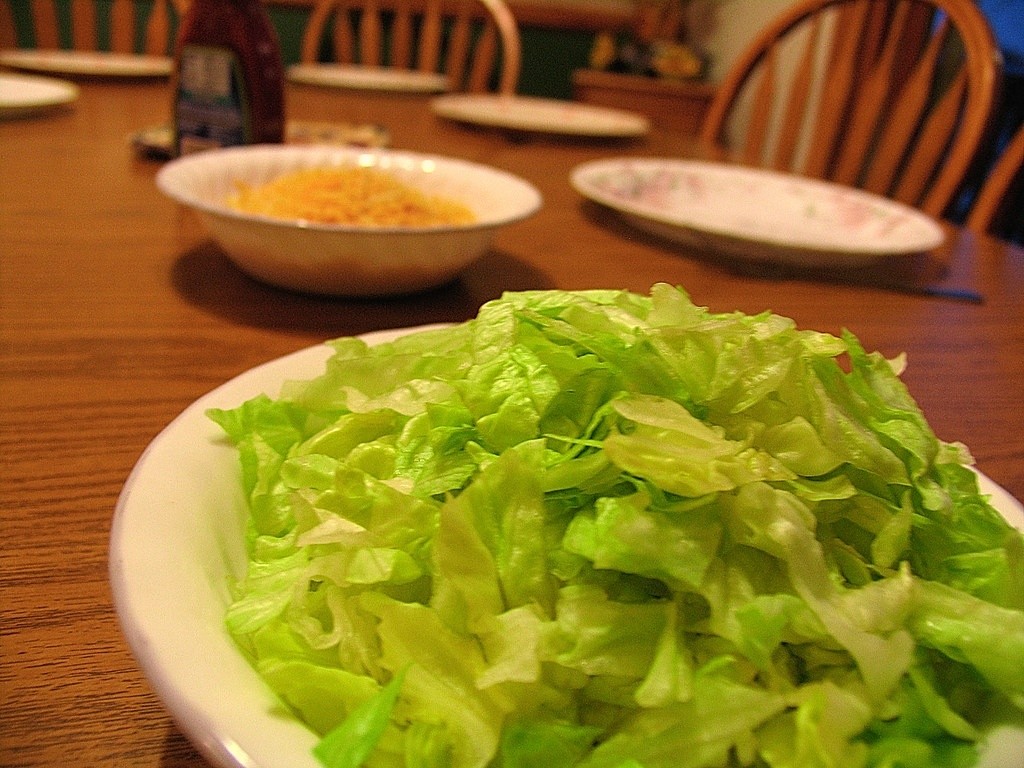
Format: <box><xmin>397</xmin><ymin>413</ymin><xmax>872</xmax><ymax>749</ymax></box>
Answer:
<box><xmin>205</xmin><ymin>282</ymin><xmax>1024</xmax><ymax>768</ymax></box>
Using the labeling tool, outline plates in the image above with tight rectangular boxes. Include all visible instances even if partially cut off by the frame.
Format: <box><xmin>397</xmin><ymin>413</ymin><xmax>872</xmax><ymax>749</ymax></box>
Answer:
<box><xmin>287</xmin><ymin>59</ymin><xmax>447</xmax><ymax>92</ymax></box>
<box><xmin>431</xmin><ymin>92</ymin><xmax>652</xmax><ymax>138</ymax></box>
<box><xmin>136</xmin><ymin>117</ymin><xmax>394</xmax><ymax>154</ymax></box>
<box><xmin>108</xmin><ymin>314</ymin><xmax>1024</xmax><ymax>768</ymax></box>
<box><xmin>0</xmin><ymin>45</ymin><xmax>173</xmax><ymax>77</ymax></box>
<box><xmin>0</xmin><ymin>72</ymin><xmax>81</xmax><ymax>119</ymax></box>
<box><xmin>567</xmin><ymin>156</ymin><xmax>946</xmax><ymax>271</ymax></box>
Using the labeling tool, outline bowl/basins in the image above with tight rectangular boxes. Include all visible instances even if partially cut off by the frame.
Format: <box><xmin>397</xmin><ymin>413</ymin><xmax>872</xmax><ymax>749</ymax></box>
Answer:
<box><xmin>156</xmin><ymin>144</ymin><xmax>543</xmax><ymax>296</ymax></box>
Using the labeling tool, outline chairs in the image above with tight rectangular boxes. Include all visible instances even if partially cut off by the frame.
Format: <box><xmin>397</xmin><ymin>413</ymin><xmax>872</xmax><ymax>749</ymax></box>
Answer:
<box><xmin>0</xmin><ymin>0</ymin><xmax>1024</xmax><ymax>243</ymax></box>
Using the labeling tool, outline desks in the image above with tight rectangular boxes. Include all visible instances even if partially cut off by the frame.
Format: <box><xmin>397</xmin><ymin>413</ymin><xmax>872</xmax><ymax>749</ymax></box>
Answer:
<box><xmin>0</xmin><ymin>60</ymin><xmax>1024</xmax><ymax>768</ymax></box>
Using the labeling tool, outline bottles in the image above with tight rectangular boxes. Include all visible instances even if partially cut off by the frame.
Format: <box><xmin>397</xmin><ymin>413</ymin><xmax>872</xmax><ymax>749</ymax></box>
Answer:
<box><xmin>174</xmin><ymin>0</ymin><xmax>293</xmax><ymax>146</ymax></box>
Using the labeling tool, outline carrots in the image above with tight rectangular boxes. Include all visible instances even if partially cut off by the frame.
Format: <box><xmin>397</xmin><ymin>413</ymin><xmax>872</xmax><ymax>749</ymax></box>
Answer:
<box><xmin>231</xmin><ymin>158</ymin><xmax>479</xmax><ymax>229</ymax></box>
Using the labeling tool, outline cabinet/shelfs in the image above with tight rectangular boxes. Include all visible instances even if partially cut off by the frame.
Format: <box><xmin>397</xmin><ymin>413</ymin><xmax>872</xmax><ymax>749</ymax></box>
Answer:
<box><xmin>575</xmin><ymin>69</ymin><xmax>712</xmax><ymax>140</ymax></box>
